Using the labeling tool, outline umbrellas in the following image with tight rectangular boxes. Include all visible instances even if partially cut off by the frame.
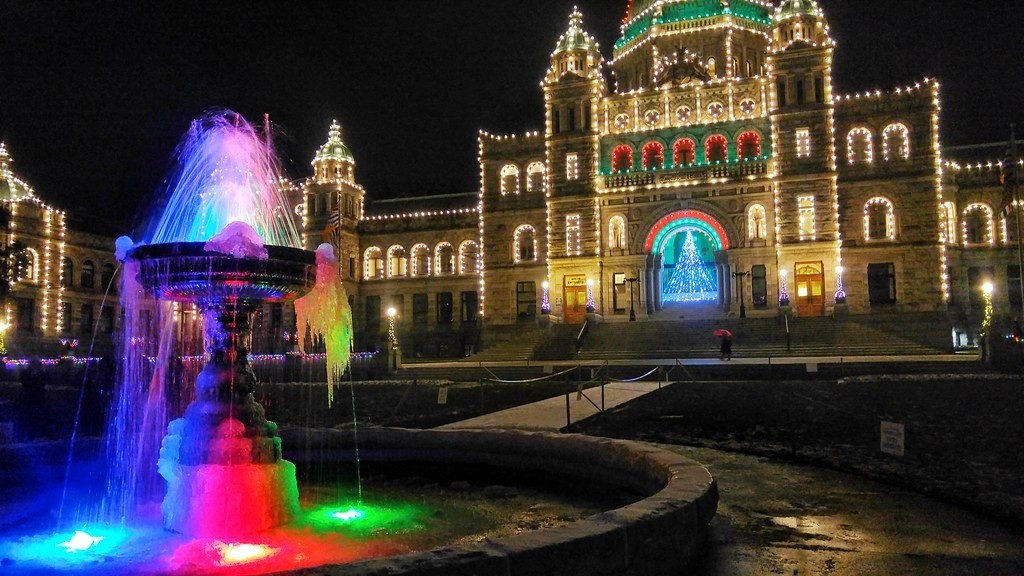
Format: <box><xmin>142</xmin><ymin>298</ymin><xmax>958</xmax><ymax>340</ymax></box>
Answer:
<box><xmin>713</xmin><ymin>329</ymin><xmax>732</xmax><ymax>337</ymax></box>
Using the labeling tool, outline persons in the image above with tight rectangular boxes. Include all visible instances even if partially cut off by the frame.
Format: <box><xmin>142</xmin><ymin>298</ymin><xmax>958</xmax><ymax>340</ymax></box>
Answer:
<box><xmin>720</xmin><ymin>332</ymin><xmax>733</xmax><ymax>361</ymax></box>
<box><xmin>0</xmin><ymin>349</ymin><xmax>116</xmax><ymax>442</ymax></box>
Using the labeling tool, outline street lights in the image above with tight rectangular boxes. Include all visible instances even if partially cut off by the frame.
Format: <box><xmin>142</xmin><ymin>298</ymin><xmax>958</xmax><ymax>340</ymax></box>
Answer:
<box><xmin>731</xmin><ymin>270</ymin><xmax>751</xmax><ymax>318</ymax></box>
<box><xmin>622</xmin><ymin>277</ymin><xmax>640</xmax><ymax>322</ymax></box>
